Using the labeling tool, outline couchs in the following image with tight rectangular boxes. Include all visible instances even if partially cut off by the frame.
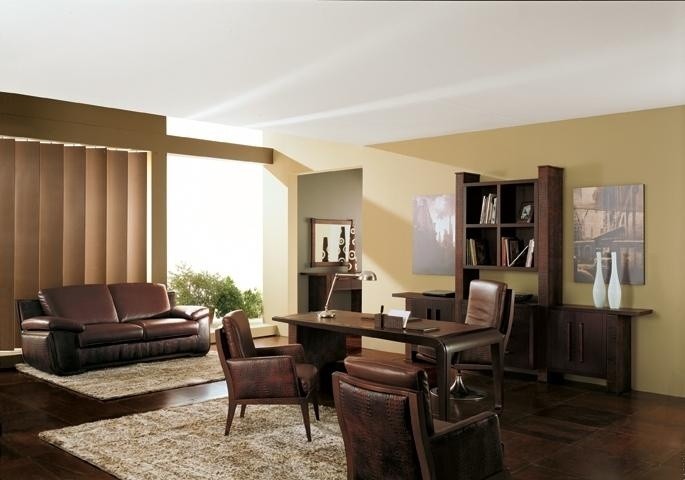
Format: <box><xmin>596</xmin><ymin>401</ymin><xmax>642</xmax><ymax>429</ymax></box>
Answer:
<box><xmin>14</xmin><ymin>282</ymin><xmax>210</xmax><ymax>376</ymax></box>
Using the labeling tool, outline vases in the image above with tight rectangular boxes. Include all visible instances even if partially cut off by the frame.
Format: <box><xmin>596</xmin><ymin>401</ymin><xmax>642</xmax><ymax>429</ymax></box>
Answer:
<box><xmin>593</xmin><ymin>252</ymin><xmax>606</xmax><ymax>308</ymax></box>
<box><xmin>608</xmin><ymin>252</ymin><xmax>622</xmax><ymax>309</ymax></box>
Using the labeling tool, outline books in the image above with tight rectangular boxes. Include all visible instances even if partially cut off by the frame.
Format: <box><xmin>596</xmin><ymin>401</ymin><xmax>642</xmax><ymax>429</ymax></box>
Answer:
<box><xmin>466</xmin><ymin>191</ymin><xmax>535</xmax><ymax>269</ymax></box>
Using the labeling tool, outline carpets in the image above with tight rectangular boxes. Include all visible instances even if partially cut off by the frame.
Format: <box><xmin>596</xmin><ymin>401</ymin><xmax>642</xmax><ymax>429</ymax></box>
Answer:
<box><xmin>38</xmin><ymin>395</ymin><xmax>348</xmax><ymax>480</ymax></box>
<box><xmin>15</xmin><ymin>351</ymin><xmax>225</xmax><ymax>400</ymax></box>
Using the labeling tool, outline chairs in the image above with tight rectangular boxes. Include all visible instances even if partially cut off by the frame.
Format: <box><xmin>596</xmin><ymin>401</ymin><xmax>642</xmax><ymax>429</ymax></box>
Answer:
<box><xmin>216</xmin><ymin>308</ymin><xmax>319</xmax><ymax>441</ymax></box>
<box><xmin>332</xmin><ymin>355</ymin><xmax>509</xmax><ymax>480</ymax></box>
<box><xmin>416</xmin><ymin>279</ymin><xmax>515</xmax><ymax>409</ymax></box>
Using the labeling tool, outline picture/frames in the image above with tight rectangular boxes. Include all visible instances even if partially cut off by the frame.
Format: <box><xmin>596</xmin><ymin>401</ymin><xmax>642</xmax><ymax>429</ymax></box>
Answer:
<box><xmin>311</xmin><ymin>217</ymin><xmax>351</xmax><ymax>268</ymax></box>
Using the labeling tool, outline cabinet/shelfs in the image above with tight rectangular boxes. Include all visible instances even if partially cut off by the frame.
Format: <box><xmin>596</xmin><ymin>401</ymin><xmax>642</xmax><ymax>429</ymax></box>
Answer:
<box><xmin>454</xmin><ymin>165</ymin><xmax>563</xmax><ymax>383</ymax></box>
<box><xmin>405</xmin><ymin>299</ymin><xmax>455</xmax><ymax>360</ymax></box>
<box><xmin>550</xmin><ymin>310</ymin><xmax>632</xmax><ymax>394</ymax></box>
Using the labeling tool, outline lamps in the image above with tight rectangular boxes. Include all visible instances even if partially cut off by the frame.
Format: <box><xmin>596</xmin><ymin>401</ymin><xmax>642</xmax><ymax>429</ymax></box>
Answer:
<box><xmin>319</xmin><ymin>270</ymin><xmax>377</xmax><ymax>317</ymax></box>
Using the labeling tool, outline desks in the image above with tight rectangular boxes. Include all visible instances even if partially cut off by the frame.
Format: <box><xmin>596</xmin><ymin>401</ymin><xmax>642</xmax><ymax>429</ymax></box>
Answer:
<box><xmin>300</xmin><ymin>271</ymin><xmax>362</xmax><ymax>312</ymax></box>
<box><xmin>272</xmin><ymin>309</ymin><xmax>508</xmax><ymax>422</ymax></box>
